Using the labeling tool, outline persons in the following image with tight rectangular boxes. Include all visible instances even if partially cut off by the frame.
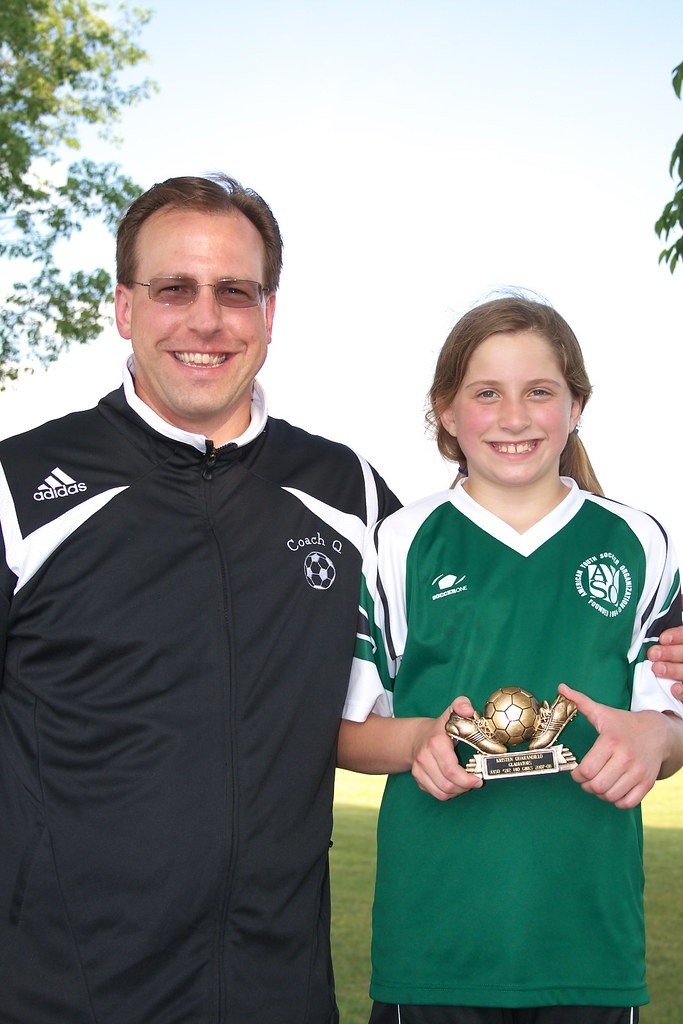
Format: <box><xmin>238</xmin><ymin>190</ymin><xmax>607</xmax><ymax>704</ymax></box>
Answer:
<box><xmin>0</xmin><ymin>172</ymin><xmax>683</xmax><ymax>1023</ymax></box>
<box><xmin>336</xmin><ymin>285</ymin><xmax>683</xmax><ymax>1024</ymax></box>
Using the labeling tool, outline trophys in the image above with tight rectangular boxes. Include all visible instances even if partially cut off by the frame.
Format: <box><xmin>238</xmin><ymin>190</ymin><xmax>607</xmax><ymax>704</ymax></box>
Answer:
<box><xmin>445</xmin><ymin>687</ymin><xmax>578</xmax><ymax>780</ymax></box>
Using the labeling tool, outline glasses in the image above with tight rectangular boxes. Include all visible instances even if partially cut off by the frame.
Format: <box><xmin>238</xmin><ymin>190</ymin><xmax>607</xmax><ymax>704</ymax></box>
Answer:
<box><xmin>124</xmin><ymin>276</ymin><xmax>276</xmax><ymax>311</ymax></box>
